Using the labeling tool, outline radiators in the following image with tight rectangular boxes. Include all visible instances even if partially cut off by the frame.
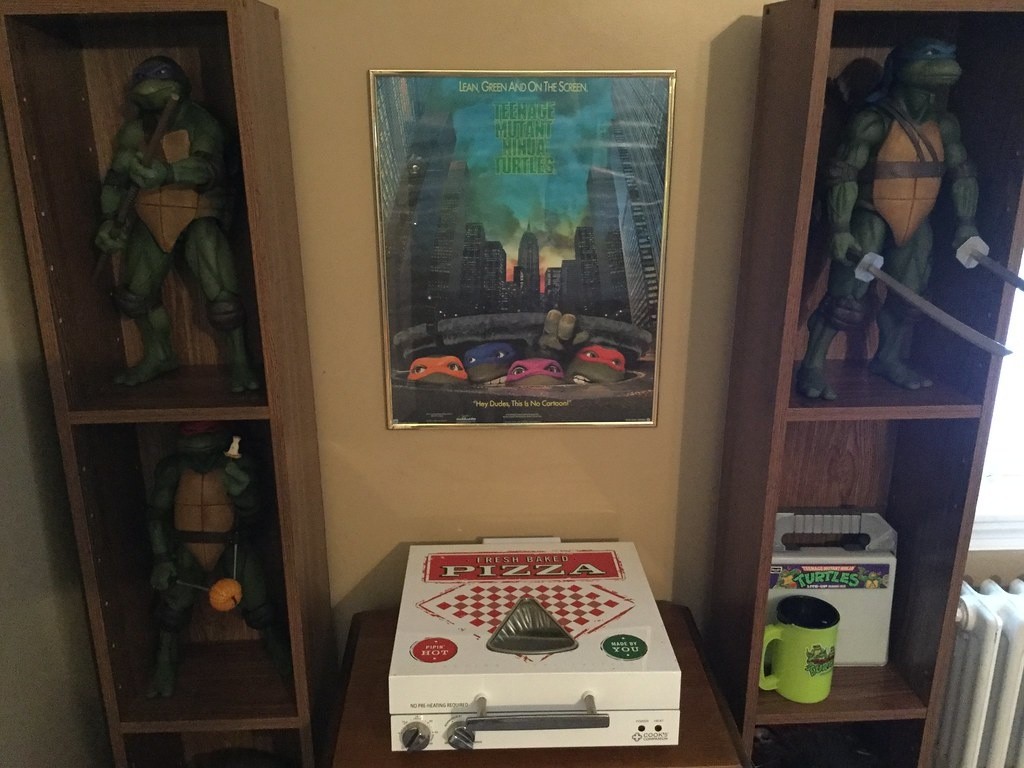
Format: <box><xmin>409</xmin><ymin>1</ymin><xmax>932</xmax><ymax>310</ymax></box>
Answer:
<box><xmin>931</xmin><ymin>578</ymin><xmax>1024</xmax><ymax>768</ymax></box>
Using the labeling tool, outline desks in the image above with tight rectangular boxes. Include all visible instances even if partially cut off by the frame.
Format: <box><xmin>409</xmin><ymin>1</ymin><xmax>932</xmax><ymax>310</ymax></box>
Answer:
<box><xmin>320</xmin><ymin>603</ymin><xmax>752</xmax><ymax>768</ymax></box>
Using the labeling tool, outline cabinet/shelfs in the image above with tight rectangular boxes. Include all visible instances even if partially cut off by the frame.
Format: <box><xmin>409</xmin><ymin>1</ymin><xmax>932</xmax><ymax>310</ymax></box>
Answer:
<box><xmin>702</xmin><ymin>1</ymin><xmax>1024</xmax><ymax>768</ymax></box>
<box><xmin>0</xmin><ymin>0</ymin><xmax>338</xmax><ymax>768</ymax></box>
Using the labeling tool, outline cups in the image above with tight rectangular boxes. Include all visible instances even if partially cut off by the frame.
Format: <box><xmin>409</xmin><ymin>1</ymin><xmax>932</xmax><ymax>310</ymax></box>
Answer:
<box><xmin>758</xmin><ymin>594</ymin><xmax>840</xmax><ymax>704</ymax></box>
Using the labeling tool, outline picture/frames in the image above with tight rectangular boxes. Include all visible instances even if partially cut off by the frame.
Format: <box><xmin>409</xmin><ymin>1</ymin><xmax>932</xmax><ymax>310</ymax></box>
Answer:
<box><xmin>367</xmin><ymin>68</ymin><xmax>680</xmax><ymax>429</ymax></box>
